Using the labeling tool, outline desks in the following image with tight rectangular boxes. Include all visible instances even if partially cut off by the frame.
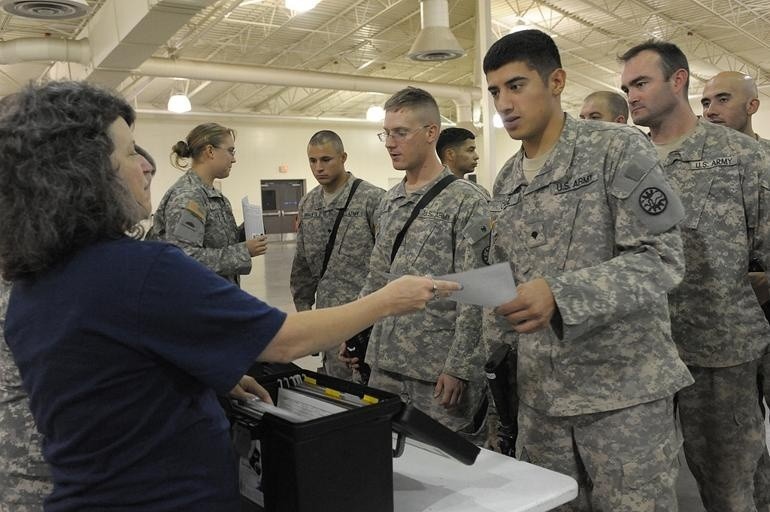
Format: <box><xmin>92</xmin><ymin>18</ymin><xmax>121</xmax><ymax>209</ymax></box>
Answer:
<box><xmin>386</xmin><ymin>429</ymin><xmax>579</xmax><ymax>511</ymax></box>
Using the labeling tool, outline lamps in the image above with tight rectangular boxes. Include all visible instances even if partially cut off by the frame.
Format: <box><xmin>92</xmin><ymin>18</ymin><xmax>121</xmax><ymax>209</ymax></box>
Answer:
<box><xmin>509</xmin><ymin>19</ymin><xmax>526</xmax><ymax>34</ymax></box>
<box><xmin>166</xmin><ymin>92</ymin><xmax>193</xmax><ymax>113</ymax></box>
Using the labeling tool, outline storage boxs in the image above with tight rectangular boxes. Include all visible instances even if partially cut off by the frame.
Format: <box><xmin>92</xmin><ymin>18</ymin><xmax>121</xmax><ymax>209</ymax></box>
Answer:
<box><xmin>218</xmin><ymin>359</ymin><xmax>481</xmax><ymax>512</ymax></box>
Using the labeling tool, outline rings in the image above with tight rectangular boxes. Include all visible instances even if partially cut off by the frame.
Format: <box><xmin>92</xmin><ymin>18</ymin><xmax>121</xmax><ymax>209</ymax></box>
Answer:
<box><xmin>431</xmin><ymin>281</ymin><xmax>437</xmax><ymax>293</ymax></box>
<box><xmin>432</xmin><ymin>292</ymin><xmax>440</xmax><ymax>302</ymax></box>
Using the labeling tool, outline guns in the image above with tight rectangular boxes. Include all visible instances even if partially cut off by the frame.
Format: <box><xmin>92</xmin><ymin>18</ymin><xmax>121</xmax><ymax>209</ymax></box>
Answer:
<box><xmin>484</xmin><ymin>342</ymin><xmax>517</xmax><ymax>458</ymax></box>
<box><xmin>345</xmin><ymin>333</ymin><xmax>371</xmax><ymax>385</ymax></box>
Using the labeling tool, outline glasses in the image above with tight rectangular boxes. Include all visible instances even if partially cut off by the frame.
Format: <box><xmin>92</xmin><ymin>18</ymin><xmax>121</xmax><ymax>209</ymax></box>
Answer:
<box><xmin>212</xmin><ymin>145</ymin><xmax>236</xmax><ymax>156</ymax></box>
<box><xmin>377</xmin><ymin>125</ymin><xmax>430</xmax><ymax>144</ymax></box>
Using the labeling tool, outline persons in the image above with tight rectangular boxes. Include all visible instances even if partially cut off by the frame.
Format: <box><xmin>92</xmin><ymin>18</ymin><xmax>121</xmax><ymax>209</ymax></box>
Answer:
<box><xmin>337</xmin><ymin>86</ymin><xmax>489</xmax><ymax>449</ymax></box>
<box><xmin>617</xmin><ymin>39</ymin><xmax>770</xmax><ymax>511</ymax></box>
<box><xmin>483</xmin><ymin>29</ymin><xmax>695</xmax><ymax>511</ymax></box>
<box><xmin>289</xmin><ymin>129</ymin><xmax>388</xmax><ymax>386</ymax></box>
<box><xmin>154</xmin><ymin>121</ymin><xmax>268</xmax><ymax>292</ymax></box>
<box><xmin>0</xmin><ymin>143</ymin><xmax>157</xmax><ymax>511</ymax></box>
<box><xmin>0</xmin><ymin>75</ymin><xmax>464</xmax><ymax>512</ymax></box>
